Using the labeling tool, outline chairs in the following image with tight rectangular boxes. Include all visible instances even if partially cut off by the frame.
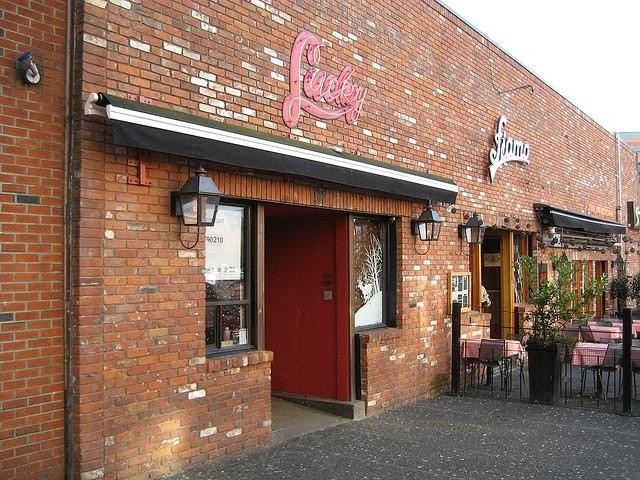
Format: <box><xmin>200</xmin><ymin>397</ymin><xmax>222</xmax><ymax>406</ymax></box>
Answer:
<box><xmin>470</xmin><ymin>312</ymin><xmax>639</xmax><ymax>411</ymax></box>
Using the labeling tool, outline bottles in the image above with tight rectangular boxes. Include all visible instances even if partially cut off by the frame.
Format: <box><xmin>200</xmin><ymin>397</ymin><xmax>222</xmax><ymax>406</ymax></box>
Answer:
<box><xmin>232</xmin><ymin>325</ymin><xmax>240</xmax><ymax>344</ymax></box>
<box><xmin>223</xmin><ymin>326</ymin><xmax>230</xmax><ymax>342</ymax></box>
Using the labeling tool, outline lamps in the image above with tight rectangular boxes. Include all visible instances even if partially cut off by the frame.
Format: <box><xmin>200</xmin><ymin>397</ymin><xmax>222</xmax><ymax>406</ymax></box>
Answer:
<box><xmin>410</xmin><ymin>199</ymin><xmax>487</xmax><ymax>255</ymax></box>
<box><xmin>170</xmin><ymin>161</ymin><xmax>222</xmax><ymax>250</ymax></box>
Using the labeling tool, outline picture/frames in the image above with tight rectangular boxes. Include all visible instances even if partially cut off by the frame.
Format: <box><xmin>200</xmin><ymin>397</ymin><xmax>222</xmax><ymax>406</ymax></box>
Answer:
<box><xmin>448</xmin><ymin>272</ymin><xmax>472</xmax><ymax>314</ymax></box>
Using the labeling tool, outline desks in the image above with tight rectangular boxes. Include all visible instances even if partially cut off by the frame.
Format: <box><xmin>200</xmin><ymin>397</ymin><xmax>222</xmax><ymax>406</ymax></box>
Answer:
<box><xmin>463</xmin><ymin>338</ymin><xmax>526</xmax><ymax>400</ymax></box>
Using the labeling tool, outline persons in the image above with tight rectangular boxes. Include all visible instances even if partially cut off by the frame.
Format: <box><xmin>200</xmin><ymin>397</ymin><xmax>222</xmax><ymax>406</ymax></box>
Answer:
<box><xmin>480</xmin><ymin>285</ymin><xmax>492</xmax><ymax>312</ymax></box>
